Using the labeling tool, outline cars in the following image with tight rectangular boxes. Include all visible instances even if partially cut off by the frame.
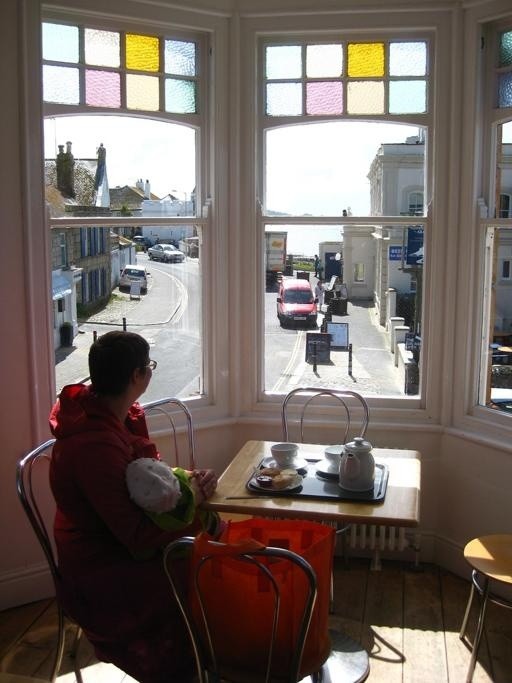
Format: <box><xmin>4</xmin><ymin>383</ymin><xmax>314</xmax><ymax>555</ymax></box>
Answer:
<box><xmin>326</xmin><ymin>448</ymin><xmax>342</xmax><ymax>464</ymax></box>
<box><xmin>271</xmin><ymin>444</ymin><xmax>299</xmax><ymax>466</ymax></box>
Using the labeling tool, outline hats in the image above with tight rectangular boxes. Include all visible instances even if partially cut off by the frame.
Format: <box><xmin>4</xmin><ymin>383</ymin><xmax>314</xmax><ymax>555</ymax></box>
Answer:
<box><xmin>147</xmin><ymin>244</ymin><xmax>185</xmax><ymax>263</ymax></box>
<box><xmin>133</xmin><ymin>236</ymin><xmax>147</xmax><ymax>253</ymax></box>
<box><xmin>118</xmin><ymin>264</ymin><xmax>150</xmax><ymax>292</ymax></box>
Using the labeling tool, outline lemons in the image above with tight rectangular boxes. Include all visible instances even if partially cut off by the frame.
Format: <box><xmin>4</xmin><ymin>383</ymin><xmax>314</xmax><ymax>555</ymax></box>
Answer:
<box><xmin>338</xmin><ymin>438</ymin><xmax>375</xmax><ymax>493</ymax></box>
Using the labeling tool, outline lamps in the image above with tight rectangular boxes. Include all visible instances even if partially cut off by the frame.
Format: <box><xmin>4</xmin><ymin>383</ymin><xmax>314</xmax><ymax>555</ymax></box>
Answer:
<box><xmin>15</xmin><ymin>398</ymin><xmax>196</xmax><ymax>683</ymax></box>
<box><xmin>458</xmin><ymin>533</ymin><xmax>512</xmax><ymax>683</ymax></box>
<box><xmin>163</xmin><ymin>537</ymin><xmax>371</xmax><ymax>683</ymax></box>
<box><xmin>281</xmin><ymin>387</ymin><xmax>370</xmax><ymax>443</ymax></box>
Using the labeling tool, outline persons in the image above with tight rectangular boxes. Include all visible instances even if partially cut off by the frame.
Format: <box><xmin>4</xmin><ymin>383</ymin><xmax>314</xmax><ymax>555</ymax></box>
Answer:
<box><xmin>121</xmin><ymin>458</ymin><xmax>226</xmax><ymax>561</ymax></box>
<box><xmin>314</xmin><ymin>255</ymin><xmax>320</xmax><ymax>277</ymax></box>
<box><xmin>314</xmin><ymin>281</ymin><xmax>324</xmax><ymax>311</ymax></box>
<box><xmin>48</xmin><ymin>330</ymin><xmax>217</xmax><ymax>683</ymax></box>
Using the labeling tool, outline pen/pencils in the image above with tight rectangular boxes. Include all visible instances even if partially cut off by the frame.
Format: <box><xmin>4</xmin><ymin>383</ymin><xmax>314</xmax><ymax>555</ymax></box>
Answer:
<box><xmin>276</xmin><ymin>278</ymin><xmax>319</xmax><ymax>326</ymax></box>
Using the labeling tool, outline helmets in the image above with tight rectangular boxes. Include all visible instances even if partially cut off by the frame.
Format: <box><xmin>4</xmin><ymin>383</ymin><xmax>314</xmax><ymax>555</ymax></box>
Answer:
<box><xmin>261</xmin><ymin>456</ymin><xmax>309</xmax><ymax>471</ymax></box>
<box><xmin>250</xmin><ymin>473</ymin><xmax>303</xmax><ymax>492</ymax></box>
<box><xmin>316</xmin><ymin>460</ymin><xmax>346</xmax><ymax>476</ymax></box>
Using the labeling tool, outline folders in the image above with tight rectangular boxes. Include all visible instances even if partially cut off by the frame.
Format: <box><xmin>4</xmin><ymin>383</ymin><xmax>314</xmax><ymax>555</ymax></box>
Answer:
<box><xmin>251</xmin><ymin>516</ymin><xmax>408</xmax><ymax>550</ymax></box>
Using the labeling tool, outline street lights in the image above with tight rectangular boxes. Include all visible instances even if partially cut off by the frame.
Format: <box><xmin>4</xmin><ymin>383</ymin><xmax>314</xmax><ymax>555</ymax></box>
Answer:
<box><xmin>189</xmin><ymin>520</ymin><xmax>335</xmax><ymax>680</ymax></box>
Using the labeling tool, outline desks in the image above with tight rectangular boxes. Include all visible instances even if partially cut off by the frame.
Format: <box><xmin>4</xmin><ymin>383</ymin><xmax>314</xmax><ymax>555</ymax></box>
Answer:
<box><xmin>171</xmin><ymin>189</ymin><xmax>188</xmax><ymax>243</ymax></box>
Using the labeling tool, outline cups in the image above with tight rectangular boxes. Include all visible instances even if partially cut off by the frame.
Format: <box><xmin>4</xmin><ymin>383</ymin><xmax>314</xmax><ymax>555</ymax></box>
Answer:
<box><xmin>141</xmin><ymin>359</ymin><xmax>158</xmax><ymax>371</ymax></box>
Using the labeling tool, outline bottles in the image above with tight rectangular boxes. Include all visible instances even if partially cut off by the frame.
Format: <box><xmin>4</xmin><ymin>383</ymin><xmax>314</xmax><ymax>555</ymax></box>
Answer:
<box><xmin>127</xmin><ymin>457</ymin><xmax>182</xmax><ymax>513</ymax></box>
